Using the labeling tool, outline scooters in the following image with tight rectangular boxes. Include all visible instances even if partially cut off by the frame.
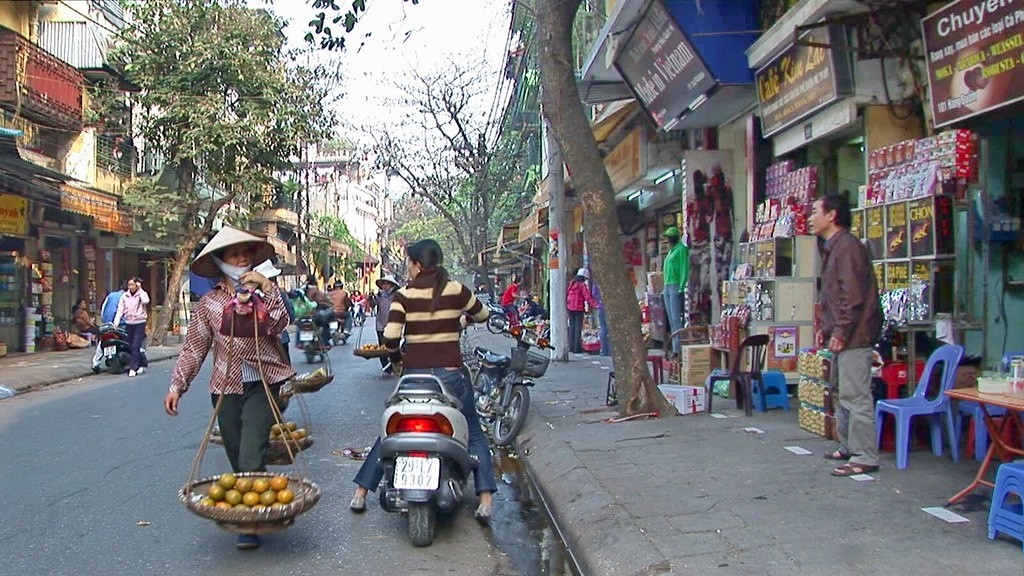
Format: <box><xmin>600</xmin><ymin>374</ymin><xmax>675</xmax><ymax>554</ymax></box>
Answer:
<box><xmin>330</xmin><ymin>300</ymin><xmax>368</xmax><ymax>347</ymax></box>
<box><xmin>96</xmin><ymin>311</ymin><xmax>149</xmax><ymax>375</ymax></box>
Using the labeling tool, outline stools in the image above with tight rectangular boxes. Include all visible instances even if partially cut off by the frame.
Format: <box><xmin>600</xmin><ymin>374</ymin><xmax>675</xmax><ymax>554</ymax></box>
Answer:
<box><xmin>78</xmin><ymin>332</ymin><xmax>97</xmax><ymax>348</ymax></box>
<box><xmin>752</xmin><ymin>372</ymin><xmax>791</xmax><ymax>412</ymax></box>
<box><xmin>966</xmin><ymin>414</ymin><xmax>1024</xmax><ymax>553</ymax></box>
<box><xmin>645</xmin><ymin>355</ymin><xmax>663</xmax><ymax>385</ymax></box>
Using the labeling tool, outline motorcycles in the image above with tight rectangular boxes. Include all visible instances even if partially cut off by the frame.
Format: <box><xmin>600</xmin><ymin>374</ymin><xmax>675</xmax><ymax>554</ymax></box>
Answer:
<box><xmin>485</xmin><ymin>291</ymin><xmax>542</xmax><ymax>335</ymax></box>
<box><xmin>377</xmin><ymin>315</ymin><xmax>484</xmax><ymax>548</ymax></box>
<box><xmin>292</xmin><ymin>305</ymin><xmax>338</xmax><ymax>365</ymax></box>
<box><xmin>468</xmin><ymin>321</ymin><xmax>556</xmax><ymax>447</ymax></box>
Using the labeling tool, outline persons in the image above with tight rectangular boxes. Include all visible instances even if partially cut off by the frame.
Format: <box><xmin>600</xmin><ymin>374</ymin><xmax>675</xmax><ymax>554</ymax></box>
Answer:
<box><xmin>73</xmin><ymin>276</ymin><xmax>150</xmax><ymax>378</ymax></box>
<box><xmin>349</xmin><ymin>238</ymin><xmax>498</xmax><ymax>521</ymax></box>
<box><xmin>254</xmin><ymin>259</ymin><xmax>600</xmax><ymax>377</ymax></box>
<box><xmin>807</xmin><ymin>190</ymin><xmax>885</xmax><ymax>475</ymax></box>
<box><xmin>164</xmin><ymin>225</ymin><xmax>297</xmax><ymax>548</ymax></box>
<box><xmin>662</xmin><ymin>227</ymin><xmax>690</xmax><ymax>356</ymax></box>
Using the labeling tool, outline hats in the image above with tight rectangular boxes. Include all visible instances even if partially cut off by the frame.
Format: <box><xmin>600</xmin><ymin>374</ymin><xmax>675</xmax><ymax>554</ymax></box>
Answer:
<box><xmin>576</xmin><ymin>268</ymin><xmax>590</xmax><ymax>279</ymax></box>
<box><xmin>375</xmin><ymin>274</ymin><xmax>401</xmax><ymax>290</ymax></box>
<box><xmin>190</xmin><ymin>226</ymin><xmax>275</xmax><ymax>279</ymax></box>
<box><xmin>307</xmin><ymin>281</ymin><xmax>317</xmax><ymax>288</ymax></box>
<box><xmin>335</xmin><ymin>280</ymin><xmax>343</xmax><ymax>287</ymax></box>
<box><xmin>662</xmin><ymin>226</ymin><xmax>680</xmax><ymax>238</ymax></box>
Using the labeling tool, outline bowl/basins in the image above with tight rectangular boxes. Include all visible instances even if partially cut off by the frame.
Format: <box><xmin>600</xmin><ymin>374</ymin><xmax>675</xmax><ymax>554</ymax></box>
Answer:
<box><xmin>978</xmin><ymin>377</ymin><xmax>1007</xmax><ymax>394</ymax></box>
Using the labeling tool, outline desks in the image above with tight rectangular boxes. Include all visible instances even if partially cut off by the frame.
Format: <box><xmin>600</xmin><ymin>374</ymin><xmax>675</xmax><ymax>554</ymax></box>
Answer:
<box><xmin>942</xmin><ymin>388</ymin><xmax>1024</xmax><ymax>506</ymax></box>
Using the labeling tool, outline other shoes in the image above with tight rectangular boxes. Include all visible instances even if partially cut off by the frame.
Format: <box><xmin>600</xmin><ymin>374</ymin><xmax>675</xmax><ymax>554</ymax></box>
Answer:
<box><xmin>297</xmin><ymin>343</ymin><xmax>304</xmax><ymax>349</ymax></box>
<box><xmin>343</xmin><ymin>329</ymin><xmax>353</xmax><ymax>336</ymax></box>
<box><xmin>351</xmin><ymin>495</ymin><xmax>364</xmax><ymax>511</ymax></box>
<box><xmin>237</xmin><ymin>533</ymin><xmax>259</xmax><ymax>548</ymax></box>
<box><xmin>318</xmin><ymin>345</ymin><xmax>331</xmax><ymax>350</ymax></box>
<box><xmin>128</xmin><ymin>367</ymin><xmax>144</xmax><ymax>377</ymax></box>
<box><xmin>474</xmin><ymin>503</ymin><xmax>492</xmax><ymax>524</ymax></box>
<box><xmin>92</xmin><ymin>365</ymin><xmax>100</xmax><ymax>374</ymax></box>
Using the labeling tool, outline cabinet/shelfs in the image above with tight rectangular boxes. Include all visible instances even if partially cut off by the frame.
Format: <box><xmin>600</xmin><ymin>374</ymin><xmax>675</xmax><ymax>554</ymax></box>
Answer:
<box><xmin>0</xmin><ymin>251</ymin><xmax>25</xmax><ymax>351</ymax></box>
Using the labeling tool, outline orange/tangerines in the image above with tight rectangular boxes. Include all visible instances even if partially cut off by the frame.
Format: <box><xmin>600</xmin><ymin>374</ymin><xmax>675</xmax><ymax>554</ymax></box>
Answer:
<box><xmin>270</xmin><ymin>422</ymin><xmax>307</xmax><ymax>440</ymax></box>
<box><xmin>200</xmin><ymin>472</ymin><xmax>293</xmax><ymax>510</ymax></box>
<box><xmin>360</xmin><ymin>344</ymin><xmax>385</xmax><ymax>350</ymax></box>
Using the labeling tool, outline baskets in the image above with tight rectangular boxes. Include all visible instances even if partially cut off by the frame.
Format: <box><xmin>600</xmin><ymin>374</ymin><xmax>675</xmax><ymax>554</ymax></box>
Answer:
<box><xmin>354</xmin><ymin>349</ymin><xmax>391</xmax><ymax>360</ymax></box>
<box><xmin>510</xmin><ymin>347</ymin><xmax>550</xmax><ymax>378</ymax></box>
<box><xmin>178</xmin><ymin>472</ymin><xmax>321</xmax><ymax>535</ymax></box>
<box><xmin>209</xmin><ymin>433</ymin><xmax>317</xmax><ymax>466</ymax></box>
<box><xmin>291</xmin><ymin>372</ymin><xmax>335</xmax><ymax>393</ymax></box>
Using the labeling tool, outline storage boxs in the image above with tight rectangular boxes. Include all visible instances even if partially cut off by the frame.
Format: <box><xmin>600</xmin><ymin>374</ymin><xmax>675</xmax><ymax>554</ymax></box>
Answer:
<box><xmin>657</xmin><ymin>345</ymin><xmax>711</xmax><ymax>415</ymax></box>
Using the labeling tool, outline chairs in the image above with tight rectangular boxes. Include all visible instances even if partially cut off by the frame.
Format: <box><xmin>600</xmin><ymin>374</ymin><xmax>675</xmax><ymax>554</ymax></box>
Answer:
<box><xmin>874</xmin><ymin>344</ymin><xmax>958</xmax><ymax>469</ymax></box>
<box><xmin>664</xmin><ymin>326</ymin><xmax>710</xmax><ymax>386</ymax></box>
<box><xmin>709</xmin><ymin>334</ymin><xmax>769</xmax><ymax>413</ymax></box>
<box><xmin>953</xmin><ymin>351</ymin><xmax>1024</xmax><ymax>462</ymax></box>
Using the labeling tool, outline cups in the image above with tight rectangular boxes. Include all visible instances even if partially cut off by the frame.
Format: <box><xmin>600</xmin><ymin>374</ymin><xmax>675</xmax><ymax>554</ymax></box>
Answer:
<box><xmin>1007</xmin><ymin>359</ymin><xmax>1024</xmax><ymax>397</ymax></box>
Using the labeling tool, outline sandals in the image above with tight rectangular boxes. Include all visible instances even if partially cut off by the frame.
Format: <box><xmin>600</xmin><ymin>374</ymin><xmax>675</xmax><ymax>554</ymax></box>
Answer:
<box><xmin>831</xmin><ymin>461</ymin><xmax>878</xmax><ymax>476</ymax></box>
<box><xmin>824</xmin><ymin>448</ymin><xmax>850</xmax><ymax>460</ymax></box>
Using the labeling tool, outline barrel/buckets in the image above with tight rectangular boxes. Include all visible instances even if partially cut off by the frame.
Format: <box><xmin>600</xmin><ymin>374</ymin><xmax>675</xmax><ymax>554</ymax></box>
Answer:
<box><xmin>24</xmin><ymin>307</ymin><xmax>36</xmax><ymax>353</ymax></box>
